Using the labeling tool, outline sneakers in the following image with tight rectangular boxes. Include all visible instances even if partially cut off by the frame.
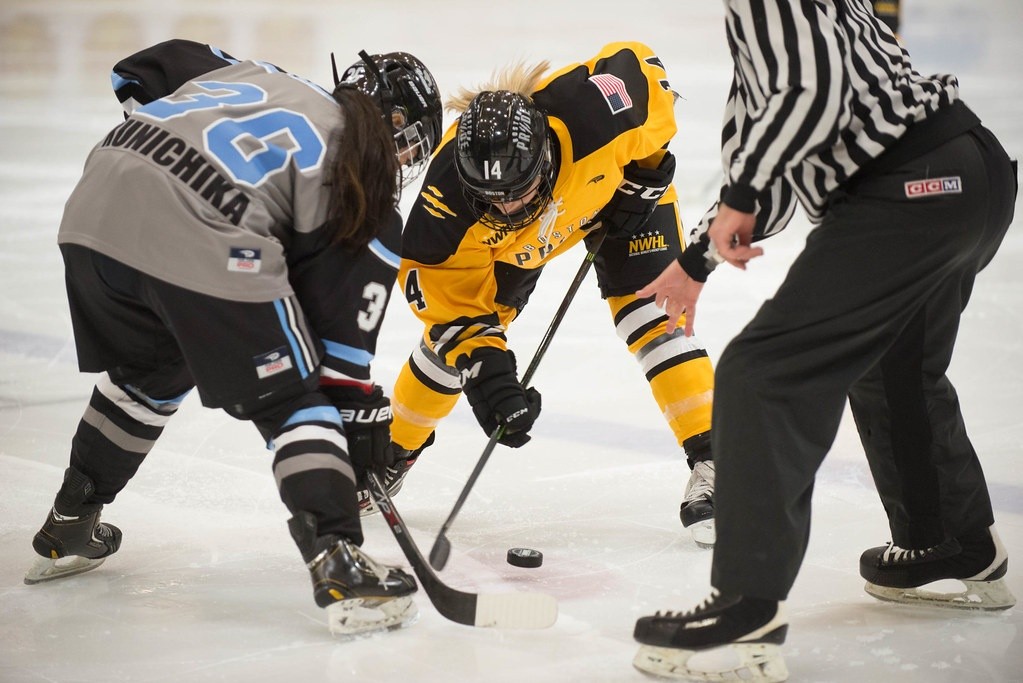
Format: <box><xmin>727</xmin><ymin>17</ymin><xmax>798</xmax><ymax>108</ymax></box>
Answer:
<box><xmin>679</xmin><ymin>457</ymin><xmax>715</xmax><ymax>546</ymax></box>
<box><xmin>288</xmin><ymin>511</ymin><xmax>420</xmax><ymax>635</ymax></box>
<box><xmin>861</xmin><ymin>517</ymin><xmax>1015</xmax><ymax>611</ymax></box>
<box><xmin>630</xmin><ymin>589</ymin><xmax>789</xmax><ymax>682</ymax></box>
<box><xmin>356</xmin><ymin>431</ymin><xmax>435</xmax><ymax>517</ymax></box>
<box><xmin>24</xmin><ymin>466</ymin><xmax>123</xmax><ymax>585</ymax></box>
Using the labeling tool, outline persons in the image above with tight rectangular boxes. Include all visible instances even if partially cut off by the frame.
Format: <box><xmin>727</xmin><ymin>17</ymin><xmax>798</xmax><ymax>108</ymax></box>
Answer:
<box><xmin>358</xmin><ymin>41</ymin><xmax>719</xmax><ymax>545</ymax></box>
<box><xmin>632</xmin><ymin>0</ymin><xmax>1019</xmax><ymax>683</ymax></box>
<box><xmin>25</xmin><ymin>39</ymin><xmax>443</xmax><ymax>637</ymax></box>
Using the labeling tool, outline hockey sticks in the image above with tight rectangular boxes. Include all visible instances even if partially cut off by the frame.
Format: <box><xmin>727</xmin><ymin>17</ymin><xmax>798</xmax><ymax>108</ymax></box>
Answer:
<box><xmin>425</xmin><ymin>226</ymin><xmax>611</xmax><ymax>574</ymax></box>
<box><xmin>363</xmin><ymin>469</ymin><xmax>518</xmax><ymax>629</ymax></box>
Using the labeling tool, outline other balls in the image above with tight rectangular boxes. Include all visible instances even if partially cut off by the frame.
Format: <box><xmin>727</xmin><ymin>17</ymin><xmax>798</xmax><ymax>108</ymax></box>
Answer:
<box><xmin>506</xmin><ymin>546</ymin><xmax>544</xmax><ymax>570</ymax></box>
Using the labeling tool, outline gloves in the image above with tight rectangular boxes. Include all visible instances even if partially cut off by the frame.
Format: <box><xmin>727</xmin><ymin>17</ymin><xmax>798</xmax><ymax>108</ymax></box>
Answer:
<box><xmin>581</xmin><ymin>153</ymin><xmax>676</xmax><ymax>240</ymax></box>
<box><xmin>320</xmin><ymin>386</ymin><xmax>395</xmax><ymax>491</ymax></box>
<box><xmin>455</xmin><ymin>346</ymin><xmax>541</xmax><ymax>449</ymax></box>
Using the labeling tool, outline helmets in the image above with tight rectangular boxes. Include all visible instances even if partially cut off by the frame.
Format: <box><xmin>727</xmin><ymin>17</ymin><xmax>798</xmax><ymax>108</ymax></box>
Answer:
<box><xmin>337</xmin><ymin>52</ymin><xmax>443</xmax><ymax>192</ymax></box>
<box><xmin>454</xmin><ymin>91</ymin><xmax>557</xmax><ymax>231</ymax></box>
